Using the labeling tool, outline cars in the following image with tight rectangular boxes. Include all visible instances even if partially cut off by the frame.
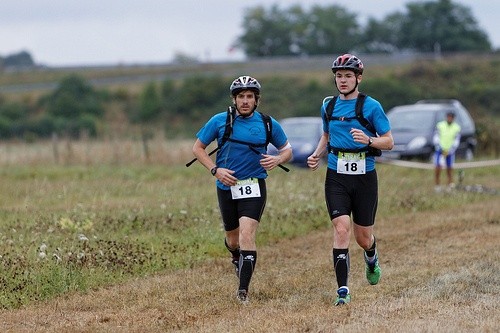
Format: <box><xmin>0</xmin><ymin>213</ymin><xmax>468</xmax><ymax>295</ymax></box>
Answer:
<box><xmin>376</xmin><ymin>99</ymin><xmax>477</xmax><ymax>161</ymax></box>
<box><xmin>266</xmin><ymin>117</ymin><xmax>323</xmax><ymax>168</ymax></box>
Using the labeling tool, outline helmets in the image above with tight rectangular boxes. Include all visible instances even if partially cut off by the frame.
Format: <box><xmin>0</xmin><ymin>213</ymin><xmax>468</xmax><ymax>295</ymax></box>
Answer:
<box><xmin>230</xmin><ymin>75</ymin><xmax>261</xmax><ymax>96</ymax></box>
<box><xmin>331</xmin><ymin>53</ymin><xmax>364</xmax><ymax>75</ymax></box>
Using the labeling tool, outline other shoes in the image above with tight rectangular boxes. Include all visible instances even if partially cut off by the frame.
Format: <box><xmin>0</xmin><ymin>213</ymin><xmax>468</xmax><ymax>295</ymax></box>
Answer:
<box><xmin>232</xmin><ymin>258</ymin><xmax>240</xmax><ymax>278</ymax></box>
<box><xmin>237</xmin><ymin>289</ymin><xmax>250</xmax><ymax>304</ymax></box>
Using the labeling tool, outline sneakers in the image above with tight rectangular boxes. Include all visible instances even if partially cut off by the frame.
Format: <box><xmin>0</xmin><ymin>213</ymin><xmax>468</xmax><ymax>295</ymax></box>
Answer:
<box><xmin>334</xmin><ymin>288</ymin><xmax>351</xmax><ymax>307</ymax></box>
<box><xmin>364</xmin><ymin>248</ymin><xmax>381</xmax><ymax>285</ymax></box>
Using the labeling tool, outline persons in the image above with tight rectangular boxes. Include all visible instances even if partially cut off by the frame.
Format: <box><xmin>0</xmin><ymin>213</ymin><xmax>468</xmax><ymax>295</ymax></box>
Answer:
<box><xmin>432</xmin><ymin>106</ymin><xmax>461</xmax><ymax>192</ymax></box>
<box><xmin>306</xmin><ymin>54</ymin><xmax>394</xmax><ymax>307</ymax></box>
<box><xmin>193</xmin><ymin>75</ymin><xmax>292</xmax><ymax>304</ymax></box>
